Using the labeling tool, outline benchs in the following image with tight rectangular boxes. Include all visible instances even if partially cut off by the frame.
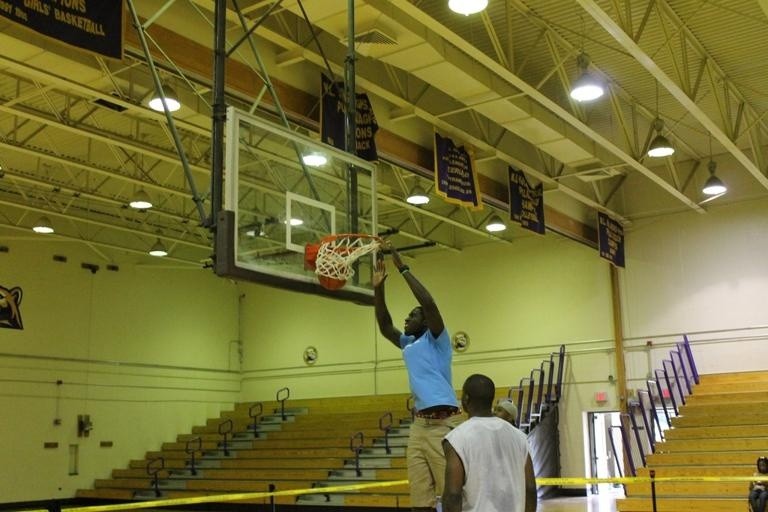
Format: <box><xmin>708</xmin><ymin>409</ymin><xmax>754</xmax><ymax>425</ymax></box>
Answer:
<box><xmin>615</xmin><ymin>370</ymin><xmax>766</xmax><ymax>511</ymax></box>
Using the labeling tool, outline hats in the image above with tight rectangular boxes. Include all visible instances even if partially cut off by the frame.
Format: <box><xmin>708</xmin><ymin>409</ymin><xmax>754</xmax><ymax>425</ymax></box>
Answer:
<box><xmin>496</xmin><ymin>400</ymin><xmax>519</xmax><ymax>428</ymax></box>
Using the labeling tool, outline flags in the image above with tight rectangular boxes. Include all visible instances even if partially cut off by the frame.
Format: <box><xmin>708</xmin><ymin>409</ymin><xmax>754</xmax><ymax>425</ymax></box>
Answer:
<box><xmin>0</xmin><ymin>1</ymin><xmax>126</xmax><ymax>66</ymax></box>
<box><xmin>317</xmin><ymin>70</ymin><xmax>379</xmax><ymax>165</ymax></box>
<box><xmin>431</xmin><ymin>123</ymin><xmax>486</xmax><ymax>213</ymax></box>
<box><xmin>504</xmin><ymin>160</ymin><xmax>546</xmax><ymax>240</ymax></box>
<box><xmin>595</xmin><ymin>206</ymin><xmax>629</xmax><ymax>271</ymax></box>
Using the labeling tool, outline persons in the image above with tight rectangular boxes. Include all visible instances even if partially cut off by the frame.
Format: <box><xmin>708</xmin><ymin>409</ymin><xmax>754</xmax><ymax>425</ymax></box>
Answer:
<box><xmin>370</xmin><ymin>237</ymin><xmax>464</xmax><ymax>511</ymax></box>
<box><xmin>490</xmin><ymin>398</ymin><xmax>519</xmax><ymax>429</ymax></box>
<box><xmin>439</xmin><ymin>374</ymin><xmax>540</xmax><ymax>511</ymax></box>
<box><xmin>747</xmin><ymin>456</ymin><xmax>768</xmax><ymax>511</ymax></box>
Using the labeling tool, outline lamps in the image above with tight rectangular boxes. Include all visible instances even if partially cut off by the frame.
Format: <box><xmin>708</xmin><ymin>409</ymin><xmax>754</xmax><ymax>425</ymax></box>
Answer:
<box><xmin>562</xmin><ymin>4</ymin><xmax>729</xmax><ymax>201</ymax></box>
<box><xmin>149</xmin><ymin>73</ymin><xmax>180</xmax><ymax>113</ymax></box>
<box><xmin>30</xmin><ymin>175</ymin><xmax>57</xmax><ymax>236</ymax></box>
<box><xmin>405</xmin><ymin>175</ymin><xmax>430</xmax><ymax>205</ymax></box>
<box><xmin>484</xmin><ymin>207</ymin><xmax>508</xmax><ymax>232</ymax></box>
<box><xmin>301</xmin><ymin>140</ymin><xmax>329</xmax><ymax>168</ymax></box>
<box><xmin>116</xmin><ymin>163</ymin><xmax>174</xmax><ymax>260</ymax></box>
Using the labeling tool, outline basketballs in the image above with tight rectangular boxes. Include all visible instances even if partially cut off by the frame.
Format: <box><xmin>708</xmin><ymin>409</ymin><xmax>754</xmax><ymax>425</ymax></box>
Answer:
<box><xmin>319</xmin><ymin>259</ymin><xmax>347</xmax><ymax>288</ymax></box>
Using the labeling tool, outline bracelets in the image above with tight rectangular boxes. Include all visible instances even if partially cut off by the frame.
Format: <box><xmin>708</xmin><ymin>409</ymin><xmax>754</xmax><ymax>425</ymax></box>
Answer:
<box><xmin>398</xmin><ymin>264</ymin><xmax>411</xmax><ymax>275</ymax></box>
